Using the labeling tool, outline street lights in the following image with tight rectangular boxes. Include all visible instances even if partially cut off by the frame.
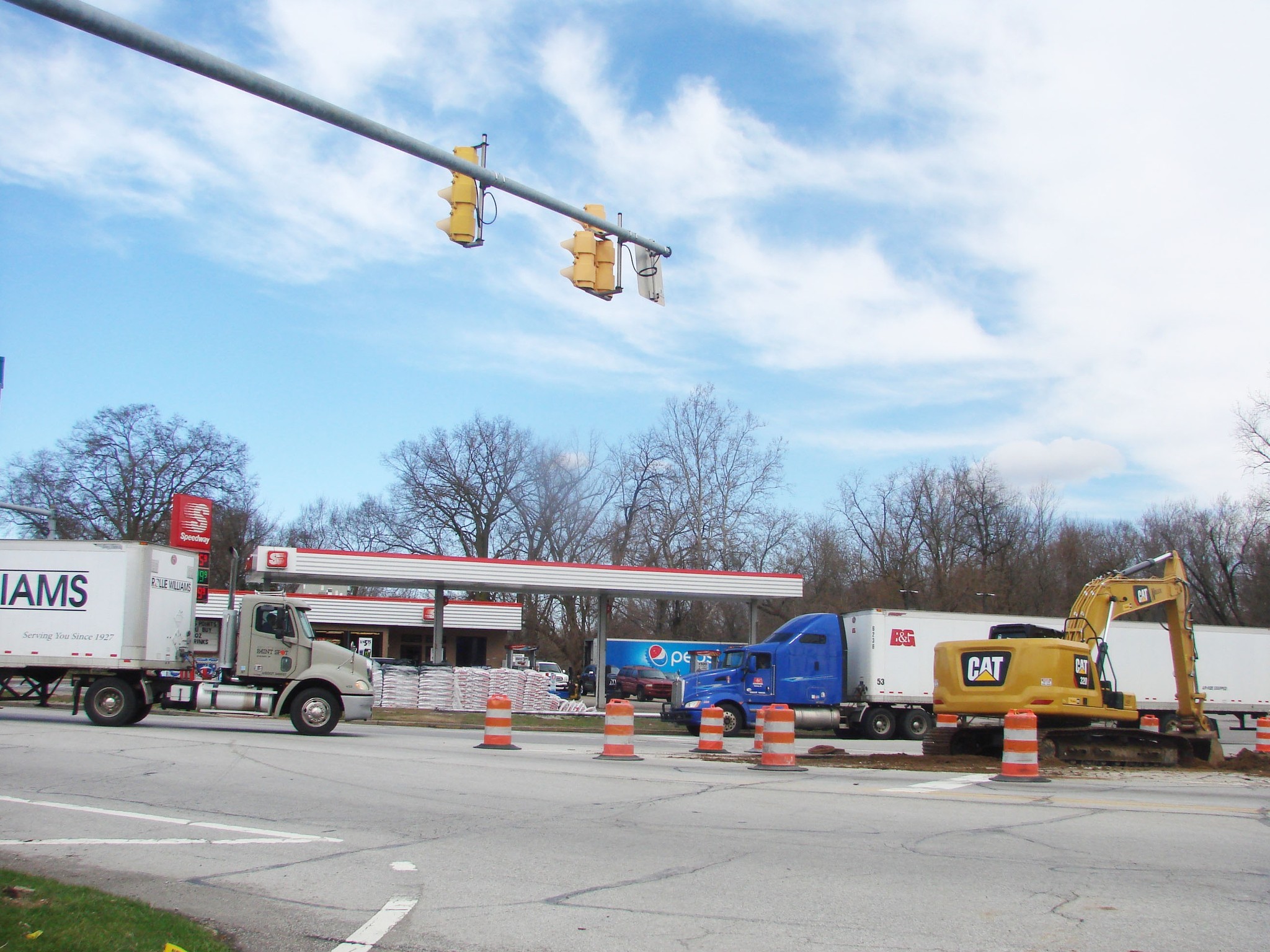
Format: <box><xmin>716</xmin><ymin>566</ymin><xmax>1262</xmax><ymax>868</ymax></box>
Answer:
<box><xmin>898</xmin><ymin>590</ymin><xmax>919</xmax><ymax>609</ymax></box>
<box><xmin>975</xmin><ymin>593</ymin><xmax>995</xmax><ymax>614</ymax></box>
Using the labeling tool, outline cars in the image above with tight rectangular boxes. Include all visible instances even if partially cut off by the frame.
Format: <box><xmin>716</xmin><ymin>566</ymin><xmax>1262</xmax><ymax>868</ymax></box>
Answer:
<box><xmin>581</xmin><ymin>665</ymin><xmax>620</xmax><ymax>695</ymax></box>
<box><xmin>537</xmin><ymin>661</ymin><xmax>568</xmax><ymax>689</ymax></box>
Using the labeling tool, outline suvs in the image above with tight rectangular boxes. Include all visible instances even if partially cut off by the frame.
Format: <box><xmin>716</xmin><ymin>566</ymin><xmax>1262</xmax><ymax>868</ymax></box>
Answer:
<box><xmin>617</xmin><ymin>665</ymin><xmax>673</xmax><ymax>701</ymax></box>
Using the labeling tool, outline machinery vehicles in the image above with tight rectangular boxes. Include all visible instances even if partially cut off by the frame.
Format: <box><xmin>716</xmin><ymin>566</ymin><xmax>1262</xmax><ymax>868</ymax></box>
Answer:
<box><xmin>922</xmin><ymin>549</ymin><xmax>1225</xmax><ymax>767</ymax></box>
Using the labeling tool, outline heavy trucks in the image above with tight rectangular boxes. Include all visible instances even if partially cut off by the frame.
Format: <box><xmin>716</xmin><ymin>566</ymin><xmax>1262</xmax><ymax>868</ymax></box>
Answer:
<box><xmin>0</xmin><ymin>538</ymin><xmax>373</xmax><ymax>734</ymax></box>
<box><xmin>659</xmin><ymin>606</ymin><xmax>1066</xmax><ymax>737</ymax></box>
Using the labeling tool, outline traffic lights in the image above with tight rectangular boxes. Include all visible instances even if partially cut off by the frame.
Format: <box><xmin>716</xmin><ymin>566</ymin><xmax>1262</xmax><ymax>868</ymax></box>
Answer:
<box><xmin>560</xmin><ymin>230</ymin><xmax>595</xmax><ymax>289</ymax></box>
<box><xmin>435</xmin><ymin>147</ymin><xmax>476</xmax><ymax>243</ymax></box>
<box><xmin>570</xmin><ymin>204</ymin><xmax>606</xmax><ymax>234</ymax></box>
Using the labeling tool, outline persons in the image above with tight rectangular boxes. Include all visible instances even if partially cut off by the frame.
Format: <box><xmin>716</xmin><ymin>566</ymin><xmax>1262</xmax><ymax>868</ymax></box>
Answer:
<box><xmin>263</xmin><ymin>613</ymin><xmax>276</xmax><ymax>633</ymax></box>
